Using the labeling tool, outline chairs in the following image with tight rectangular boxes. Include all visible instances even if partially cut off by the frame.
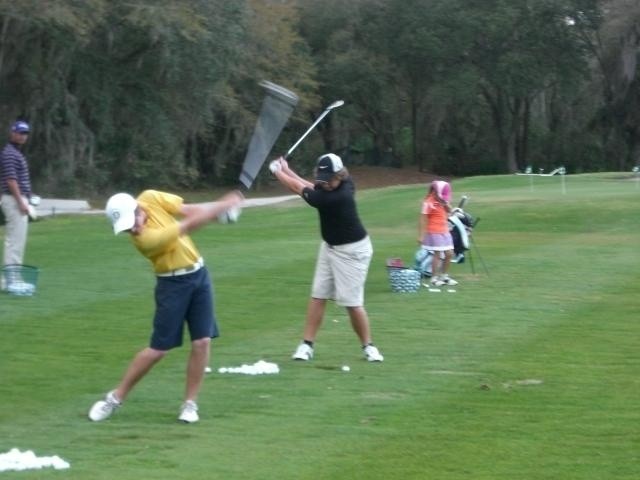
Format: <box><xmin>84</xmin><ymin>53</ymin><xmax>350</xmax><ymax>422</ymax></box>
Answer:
<box><xmin>411</xmin><ymin>192</ymin><xmax>491</xmax><ymax>278</ymax></box>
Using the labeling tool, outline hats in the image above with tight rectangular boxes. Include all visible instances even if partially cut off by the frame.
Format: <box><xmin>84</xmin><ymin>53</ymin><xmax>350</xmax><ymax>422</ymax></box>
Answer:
<box><xmin>432</xmin><ymin>181</ymin><xmax>451</xmax><ymax>202</ymax></box>
<box><xmin>314</xmin><ymin>153</ymin><xmax>343</xmax><ymax>182</ymax></box>
<box><xmin>105</xmin><ymin>192</ymin><xmax>137</xmax><ymax>235</ymax></box>
<box><xmin>12</xmin><ymin>121</ymin><xmax>29</xmax><ymax>134</ymax></box>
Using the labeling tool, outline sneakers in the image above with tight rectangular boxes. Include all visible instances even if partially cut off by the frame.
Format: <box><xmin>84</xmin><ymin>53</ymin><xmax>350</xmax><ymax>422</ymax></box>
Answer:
<box><xmin>444</xmin><ymin>275</ymin><xmax>456</xmax><ymax>285</ymax></box>
<box><xmin>89</xmin><ymin>391</ymin><xmax>120</xmax><ymax>422</ymax></box>
<box><xmin>430</xmin><ymin>277</ymin><xmax>444</xmax><ymax>286</ymax></box>
<box><xmin>293</xmin><ymin>344</ymin><xmax>314</xmax><ymax>360</ymax></box>
<box><xmin>179</xmin><ymin>400</ymin><xmax>199</xmax><ymax>423</ymax></box>
<box><xmin>365</xmin><ymin>345</ymin><xmax>383</xmax><ymax>361</ymax></box>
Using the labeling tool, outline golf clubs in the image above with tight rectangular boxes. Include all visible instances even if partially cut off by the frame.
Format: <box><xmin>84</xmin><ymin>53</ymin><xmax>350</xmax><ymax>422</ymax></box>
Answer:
<box><xmin>265</xmin><ymin>100</ymin><xmax>344</xmax><ymax>181</ymax></box>
<box><xmin>213</xmin><ymin>79</ymin><xmax>300</xmax><ymax>223</ymax></box>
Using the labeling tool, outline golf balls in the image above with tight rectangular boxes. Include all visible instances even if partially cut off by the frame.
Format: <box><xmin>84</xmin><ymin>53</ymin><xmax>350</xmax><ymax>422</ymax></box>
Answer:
<box><xmin>203</xmin><ymin>359</ymin><xmax>281</xmax><ymax>377</ymax></box>
<box><xmin>0</xmin><ymin>446</ymin><xmax>70</xmax><ymax>471</ymax></box>
<box><xmin>387</xmin><ymin>264</ymin><xmax>422</xmax><ymax>295</ymax></box>
<box><xmin>2</xmin><ymin>279</ymin><xmax>35</xmax><ymax>298</ymax></box>
<box><xmin>342</xmin><ymin>364</ymin><xmax>351</xmax><ymax>371</ymax></box>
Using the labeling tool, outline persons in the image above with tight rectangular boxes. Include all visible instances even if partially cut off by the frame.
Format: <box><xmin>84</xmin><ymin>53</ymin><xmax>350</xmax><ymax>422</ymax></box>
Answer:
<box><xmin>268</xmin><ymin>152</ymin><xmax>385</xmax><ymax>362</ymax></box>
<box><xmin>87</xmin><ymin>187</ymin><xmax>248</xmax><ymax>422</ymax></box>
<box><xmin>0</xmin><ymin>120</ymin><xmax>41</xmax><ymax>296</ymax></box>
<box><xmin>416</xmin><ymin>180</ymin><xmax>459</xmax><ymax>286</ymax></box>
